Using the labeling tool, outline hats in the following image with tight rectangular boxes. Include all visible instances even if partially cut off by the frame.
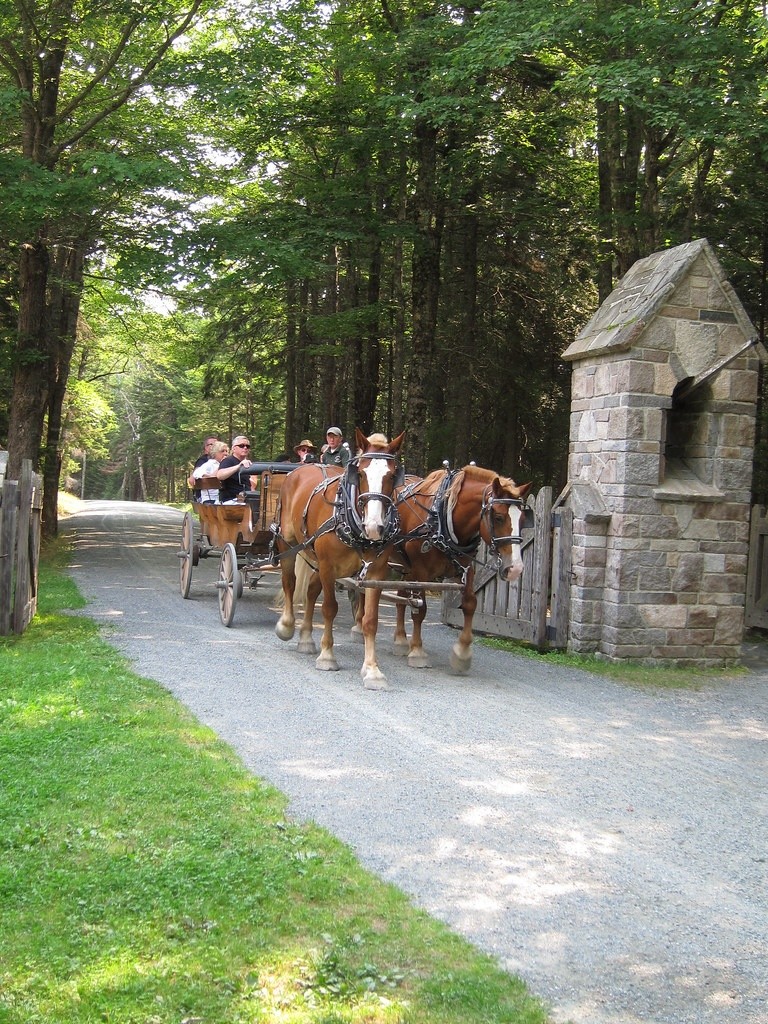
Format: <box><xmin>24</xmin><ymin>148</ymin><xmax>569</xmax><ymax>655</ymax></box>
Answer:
<box><xmin>326</xmin><ymin>426</ymin><xmax>343</xmax><ymax>436</ymax></box>
<box><xmin>366</xmin><ymin>433</ymin><xmax>390</xmax><ymax>447</ymax></box>
<box><xmin>293</xmin><ymin>439</ymin><xmax>317</xmax><ymax>454</ymax></box>
<box><xmin>320</xmin><ymin>444</ymin><xmax>330</xmax><ymax>454</ymax></box>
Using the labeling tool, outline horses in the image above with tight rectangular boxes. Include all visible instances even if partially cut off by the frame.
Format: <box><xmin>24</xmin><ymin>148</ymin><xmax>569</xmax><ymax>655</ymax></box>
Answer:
<box><xmin>274</xmin><ymin>427</ymin><xmax>533</xmax><ymax>692</ymax></box>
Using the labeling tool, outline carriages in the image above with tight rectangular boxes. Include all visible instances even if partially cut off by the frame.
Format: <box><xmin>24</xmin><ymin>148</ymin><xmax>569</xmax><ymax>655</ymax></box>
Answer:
<box><xmin>176</xmin><ymin>427</ymin><xmax>535</xmax><ymax>690</ymax></box>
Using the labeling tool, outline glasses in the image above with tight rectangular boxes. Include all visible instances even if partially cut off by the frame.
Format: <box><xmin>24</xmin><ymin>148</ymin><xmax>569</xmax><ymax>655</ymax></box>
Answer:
<box><xmin>235</xmin><ymin>444</ymin><xmax>251</xmax><ymax>449</ymax></box>
<box><xmin>301</xmin><ymin>448</ymin><xmax>310</xmax><ymax>453</ymax></box>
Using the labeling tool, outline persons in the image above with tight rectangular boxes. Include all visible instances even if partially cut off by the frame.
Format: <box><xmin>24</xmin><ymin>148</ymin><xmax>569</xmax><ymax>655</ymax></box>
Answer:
<box><xmin>320</xmin><ymin>427</ymin><xmax>352</xmax><ymax>468</ymax></box>
<box><xmin>275</xmin><ymin>455</ymin><xmax>291</xmax><ymax>463</ymax></box>
<box><xmin>216</xmin><ymin>436</ymin><xmax>252</xmax><ymax>505</ymax></box>
<box><xmin>189</xmin><ymin>436</ymin><xmax>221</xmax><ymax>501</ymax></box>
<box><xmin>192</xmin><ymin>441</ymin><xmax>229</xmax><ymax>504</ymax></box>
<box><xmin>293</xmin><ymin>440</ymin><xmax>318</xmax><ymax>464</ymax></box>
